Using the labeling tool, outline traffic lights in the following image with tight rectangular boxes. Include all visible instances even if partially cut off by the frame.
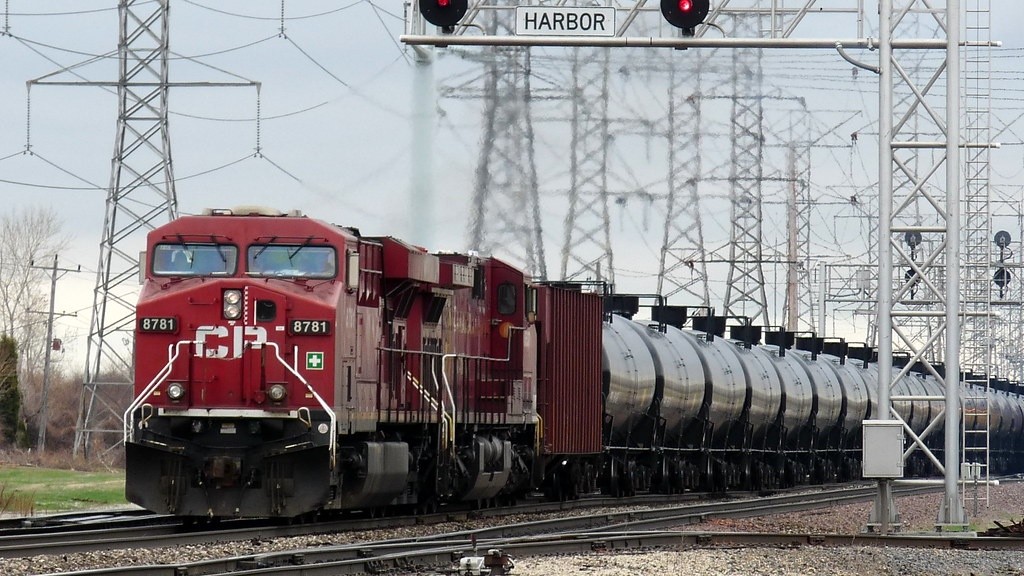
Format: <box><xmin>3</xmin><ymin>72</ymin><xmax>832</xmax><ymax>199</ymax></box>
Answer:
<box><xmin>661</xmin><ymin>0</ymin><xmax>710</xmax><ymax>28</ymax></box>
<box><xmin>419</xmin><ymin>0</ymin><xmax>468</xmax><ymax>28</ymax></box>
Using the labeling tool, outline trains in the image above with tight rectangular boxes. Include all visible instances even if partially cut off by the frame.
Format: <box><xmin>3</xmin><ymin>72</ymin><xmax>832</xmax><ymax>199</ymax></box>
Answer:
<box><xmin>120</xmin><ymin>202</ymin><xmax>1024</xmax><ymax>523</ymax></box>
